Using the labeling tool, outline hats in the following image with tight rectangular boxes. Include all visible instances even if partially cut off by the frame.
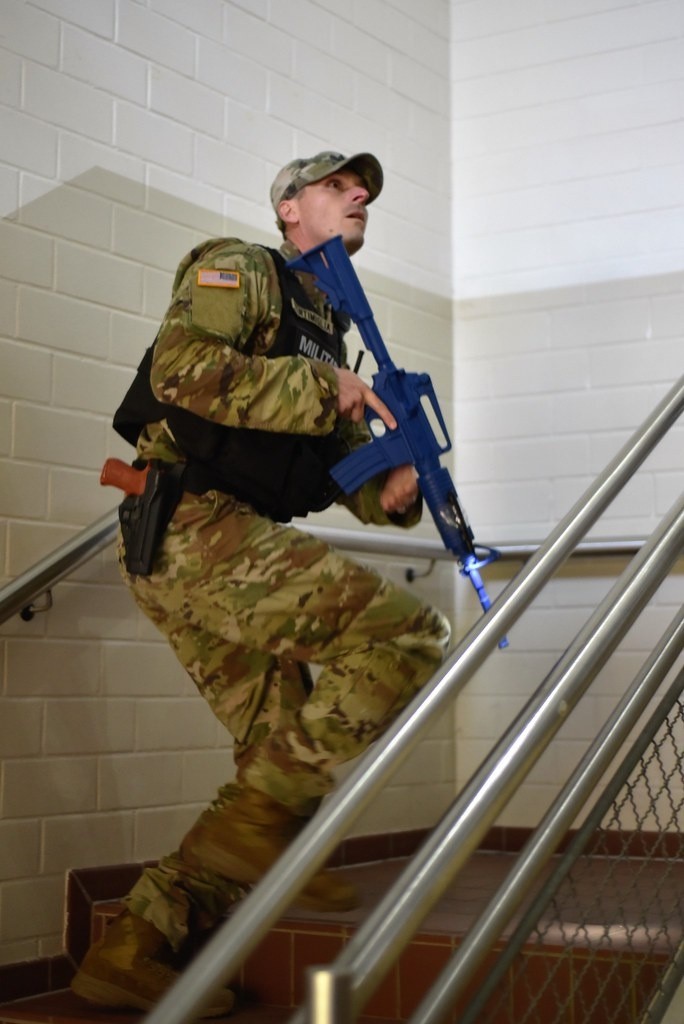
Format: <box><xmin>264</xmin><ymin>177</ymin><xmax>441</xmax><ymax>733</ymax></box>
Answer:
<box><xmin>270</xmin><ymin>150</ymin><xmax>383</xmax><ymax>229</ymax></box>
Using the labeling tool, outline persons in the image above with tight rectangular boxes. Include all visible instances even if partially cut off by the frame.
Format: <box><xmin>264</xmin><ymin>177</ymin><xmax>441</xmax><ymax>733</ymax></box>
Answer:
<box><xmin>70</xmin><ymin>150</ymin><xmax>451</xmax><ymax>1020</ymax></box>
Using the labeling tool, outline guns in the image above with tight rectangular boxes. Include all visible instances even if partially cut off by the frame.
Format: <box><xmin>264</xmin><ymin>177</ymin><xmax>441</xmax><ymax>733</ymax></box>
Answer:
<box><xmin>287</xmin><ymin>235</ymin><xmax>511</xmax><ymax>650</ymax></box>
<box><xmin>99</xmin><ymin>456</ymin><xmax>169</xmax><ymax>576</ymax></box>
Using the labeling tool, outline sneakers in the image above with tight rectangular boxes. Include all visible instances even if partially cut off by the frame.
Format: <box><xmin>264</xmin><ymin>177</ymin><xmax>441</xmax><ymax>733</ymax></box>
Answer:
<box><xmin>69</xmin><ymin>906</ymin><xmax>236</xmax><ymax>1017</ymax></box>
<box><xmin>181</xmin><ymin>784</ymin><xmax>362</xmax><ymax>913</ymax></box>
